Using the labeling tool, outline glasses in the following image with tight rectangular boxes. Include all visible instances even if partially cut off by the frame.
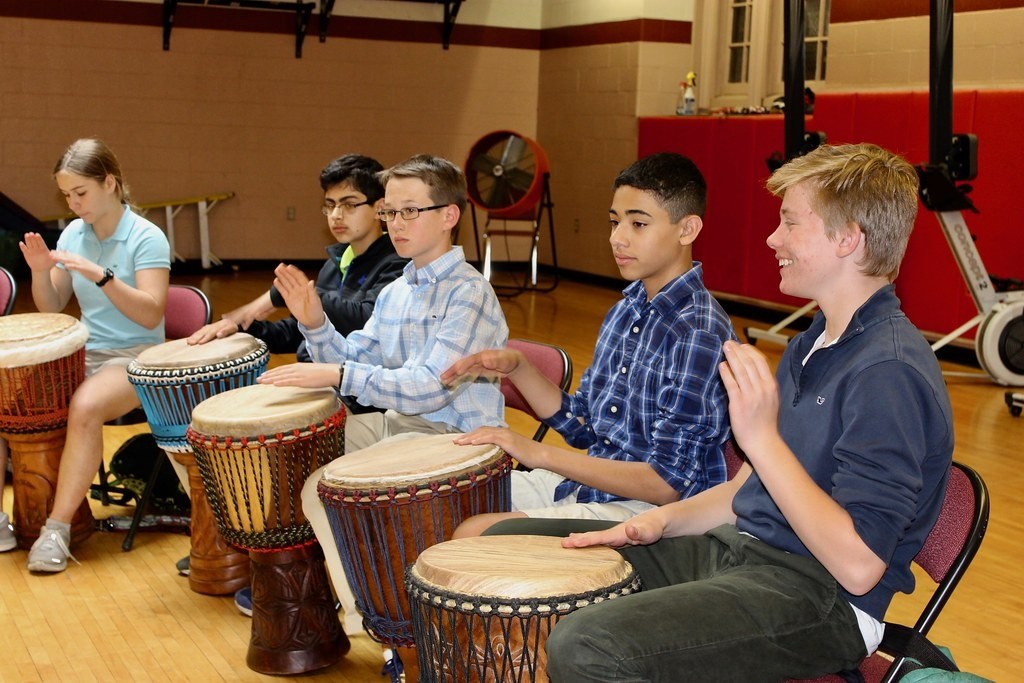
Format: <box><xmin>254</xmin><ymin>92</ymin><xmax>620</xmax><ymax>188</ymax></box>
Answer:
<box><xmin>321</xmin><ymin>201</ymin><xmax>376</xmax><ymax>215</ymax></box>
<box><xmin>378</xmin><ymin>204</ymin><xmax>449</xmax><ymax>221</ymax></box>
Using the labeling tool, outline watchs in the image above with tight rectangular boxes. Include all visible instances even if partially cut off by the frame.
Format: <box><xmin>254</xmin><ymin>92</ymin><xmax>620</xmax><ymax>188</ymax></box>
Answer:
<box><xmin>96</xmin><ymin>267</ymin><xmax>114</xmax><ymax>287</ymax></box>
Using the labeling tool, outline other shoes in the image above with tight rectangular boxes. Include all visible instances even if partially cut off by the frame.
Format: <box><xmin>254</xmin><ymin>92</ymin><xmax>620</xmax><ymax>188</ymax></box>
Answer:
<box><xmin>382</xmin><ymin>649</ymin><xmax>405</xmax><ymax>683</ymax></box>
<box><xmin>235</xmin><ymin>586</ymin><xmax>253</xmax><ymax>616</ymax></box>
<box><xmin>177</xmin><ymin>555</ymin><xmax>189</xmax><ymax>575</ymax></box>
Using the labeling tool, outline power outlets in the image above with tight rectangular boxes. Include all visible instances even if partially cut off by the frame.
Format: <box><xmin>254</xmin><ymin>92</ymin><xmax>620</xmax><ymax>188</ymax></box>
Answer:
<box><xmin>286</xmin><ymin>206</ymin><xmax>296</xmax><ymax>220</ymax></box>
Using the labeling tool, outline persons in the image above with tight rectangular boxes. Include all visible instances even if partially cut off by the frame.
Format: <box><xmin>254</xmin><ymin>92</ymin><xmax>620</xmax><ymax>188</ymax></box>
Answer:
<box><xmin>0</xmin><ymin>138</ymin><xmax>171</xmax><ymax>572</ymax></box>
<box><xmin>481</xmin><ymin>143</ymin><xmax>957</xmax><ymax>683</ymax></box>
<box><xmin>175</xmin><ymin>154</ymin><xmax>411</xmax><ymax>576</ymax></box>
<box><xmin>439</xmin><ymin>152</ymin><xmax>740</xmax><ymax>540</ymax></box>
<box><xmin>234</xmin><ymin>154</ymin><xmax>511</xmax><ymax>683</ymax></box>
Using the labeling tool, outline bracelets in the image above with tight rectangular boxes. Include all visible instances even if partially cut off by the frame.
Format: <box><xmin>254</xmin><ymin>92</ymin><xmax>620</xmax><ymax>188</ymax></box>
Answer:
<box><xmin>338</xmin><ymin>362</ymin><xmax>346</xmax><ymax>390</ymax></box>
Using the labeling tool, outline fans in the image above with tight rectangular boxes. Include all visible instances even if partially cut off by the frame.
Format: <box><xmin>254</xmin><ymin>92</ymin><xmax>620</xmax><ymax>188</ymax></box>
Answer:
<box><xmin>454</xmin><ymin>129</ymin><xmax>561</xmax><ymax>300</ymax></box>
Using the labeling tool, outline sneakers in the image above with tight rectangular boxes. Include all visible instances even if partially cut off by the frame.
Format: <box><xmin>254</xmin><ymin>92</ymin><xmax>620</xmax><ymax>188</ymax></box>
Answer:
<box><xmin>0</xmin><ymin>513</ymin><xmax>17</xmax><ymax>553</ymax></box>
<box><xmin>28</xmin><ymin>518</ymin><xmax>80</xmax><ymax>572</ymax></box>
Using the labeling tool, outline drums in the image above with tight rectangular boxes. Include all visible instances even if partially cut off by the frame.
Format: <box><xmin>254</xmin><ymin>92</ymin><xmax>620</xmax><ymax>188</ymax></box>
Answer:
<box><xmin>124</xmin><ymin>328</ymin><xmax>273</xmax><ymax>596</ymax></box>
<box><xmin>314</xmin><ymin>434</ymin><xmax>513</xmax><ymax>683</ymax></box>
<box><xmin>403</xmin><ymin>534</ymin><xmax>644</xmax><ymax>683</ymax></box>
<box><xmin>0</xmin><ymin>311</ymin><xmax>97</xmax><ymax>548</ymax></box>
<box><xmin>185</xmin><ymin>381</ymin><xmax>353</xmax><ymax>677</ymax></box>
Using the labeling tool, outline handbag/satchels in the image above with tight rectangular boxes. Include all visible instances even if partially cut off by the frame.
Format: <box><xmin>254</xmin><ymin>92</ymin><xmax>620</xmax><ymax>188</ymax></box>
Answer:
<box><xmin>90</xmin><ymin>433</ymin><xmax>189</xmax><ymax>513</ymax></box>
<box><xmin>841</xmin><ymin>624</ymin><xmax>996</xmax><ymax>683</ymax></box>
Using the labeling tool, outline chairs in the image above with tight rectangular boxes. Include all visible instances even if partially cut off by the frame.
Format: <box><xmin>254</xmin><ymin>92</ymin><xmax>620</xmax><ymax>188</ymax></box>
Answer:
<box><xmin>334</xmin><ymin>335</ymin><xmax>573</xmax><ymax>611</ymax></box>
<box><xmin>90</xmin><ymin>284</ymin><xmax>213</xmax><ymax>553</ymax></box>
<box><xmin>785</xmin><ymin>461</ymin><xmax>991</xmax><ymax>683</ymax></box>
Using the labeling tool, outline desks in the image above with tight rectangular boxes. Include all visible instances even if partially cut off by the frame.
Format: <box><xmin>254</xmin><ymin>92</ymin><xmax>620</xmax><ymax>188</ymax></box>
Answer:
<box><xmin>39</xmin><ymin>189</ymin><xmax>240</xmax><ymax>277</ymax></box>
